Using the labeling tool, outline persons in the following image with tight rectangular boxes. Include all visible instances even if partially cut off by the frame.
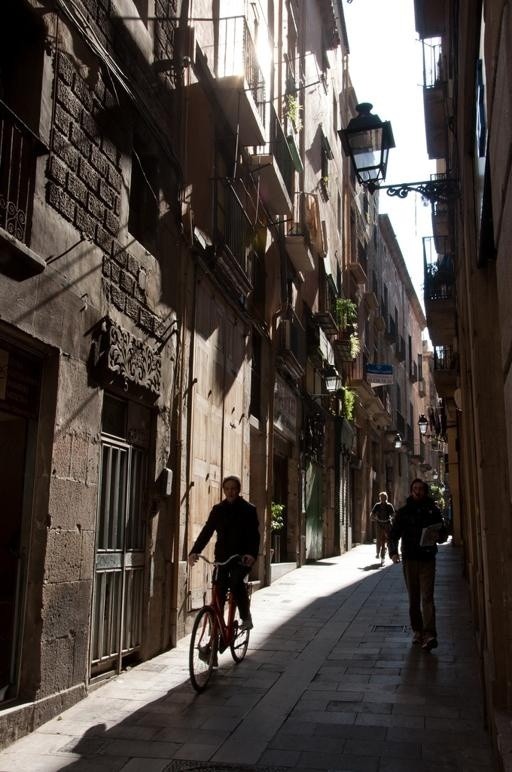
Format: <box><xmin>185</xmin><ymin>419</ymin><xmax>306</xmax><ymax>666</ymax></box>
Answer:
<box><xmin>186</xmin><ymin>475</ymin><xmax>261</xmax><ymax>666</ymax></box>
<box><xmin>369</xmin><ymin>491</ymin><xmax>396</xmax><ymax>559</ymax></box>
<box><xmin>386</xmin><ymin>477</ymin><xmax>448</xmax><ymax>651</ymax></box>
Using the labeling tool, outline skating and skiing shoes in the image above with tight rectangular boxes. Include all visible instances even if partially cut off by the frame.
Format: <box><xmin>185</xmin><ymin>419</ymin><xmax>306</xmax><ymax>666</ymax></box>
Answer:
<box><xmin>239</xmin><ymin>621</ymin><xmax>254</xmax><ymax>629</ymax></box>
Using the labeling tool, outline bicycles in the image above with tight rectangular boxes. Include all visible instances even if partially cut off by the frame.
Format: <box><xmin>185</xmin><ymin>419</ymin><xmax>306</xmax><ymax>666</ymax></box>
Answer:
<box><xmin>373</xmin><ymin>516</ymin><xmax>393</xmax><ymax>566</ymax></box>
<box><xmin>189</xmin><ymin>552</ymin><xmax>254</xmax><ymax>695</ymax></box>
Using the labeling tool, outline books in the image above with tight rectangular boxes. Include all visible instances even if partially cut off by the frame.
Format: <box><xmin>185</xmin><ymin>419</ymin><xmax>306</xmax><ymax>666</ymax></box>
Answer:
<box><xmin>420</xmin><ymin>523</ymin><xmax>442</xmax><ymax>547</ymax></box>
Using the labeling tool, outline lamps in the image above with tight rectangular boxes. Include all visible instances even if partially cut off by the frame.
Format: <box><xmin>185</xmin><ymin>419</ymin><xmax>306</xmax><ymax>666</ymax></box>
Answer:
<box><xmin>307</xmin><ymin>363</ymin><xmax>342</xmax><ymax>401</ymax></box>
<box><xmin>336</xmin><ymin>101</ymin><xmax>460</xmax><ymax>209</ymax></box>
<box><xmin>417</xmin><ymin>413</ymin><xmax>448</xmax><ymax>444</ymax></box>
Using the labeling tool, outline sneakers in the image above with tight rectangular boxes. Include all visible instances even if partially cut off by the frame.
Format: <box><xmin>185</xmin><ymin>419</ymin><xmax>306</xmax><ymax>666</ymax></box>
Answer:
<box><xmin>412</xmin><ymin>633</ymin><xmax>439</xmax><ymax>653</ymax></box>
<box><xmin>198</xmin><ymin>647</ymin><xmax>219</xmax><ymax>667</ymax></box>
